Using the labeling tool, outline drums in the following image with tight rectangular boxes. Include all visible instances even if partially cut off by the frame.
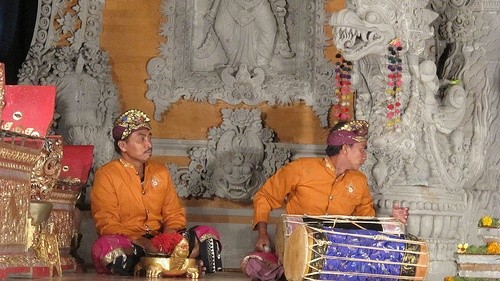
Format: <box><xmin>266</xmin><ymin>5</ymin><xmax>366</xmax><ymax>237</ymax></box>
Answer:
<box><xmin>282</xmin><ymin>224</ymin><xmax>431</xmax><ymax>281</ymax></box>
<box><xmin>274</xmin><ymin>213</ymin><xmax>406</xmax><ymax>266</ymax></box>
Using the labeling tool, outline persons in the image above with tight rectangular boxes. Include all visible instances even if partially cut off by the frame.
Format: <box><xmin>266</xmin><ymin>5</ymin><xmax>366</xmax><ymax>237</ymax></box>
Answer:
<box><xmin>91</xmin><ymin>109</ymin><xmax>224</xmax><ymax>276</ymax></box>
<box><xmin>241</xmin><ymin>120</ymin><xmax>410</xmax><ymax>281</ymax></box>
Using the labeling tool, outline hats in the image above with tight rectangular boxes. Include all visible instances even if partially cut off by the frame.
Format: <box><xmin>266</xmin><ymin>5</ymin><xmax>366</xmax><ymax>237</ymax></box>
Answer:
<box><xmin>326</xmin><ymin>120</ymin><xmax>370</xmax><ymax>146</ymax></box>
<box><xmin>109</xmin><ymin>109</ymin><xmax>152</xmax><ymax>139</ymax></box>
